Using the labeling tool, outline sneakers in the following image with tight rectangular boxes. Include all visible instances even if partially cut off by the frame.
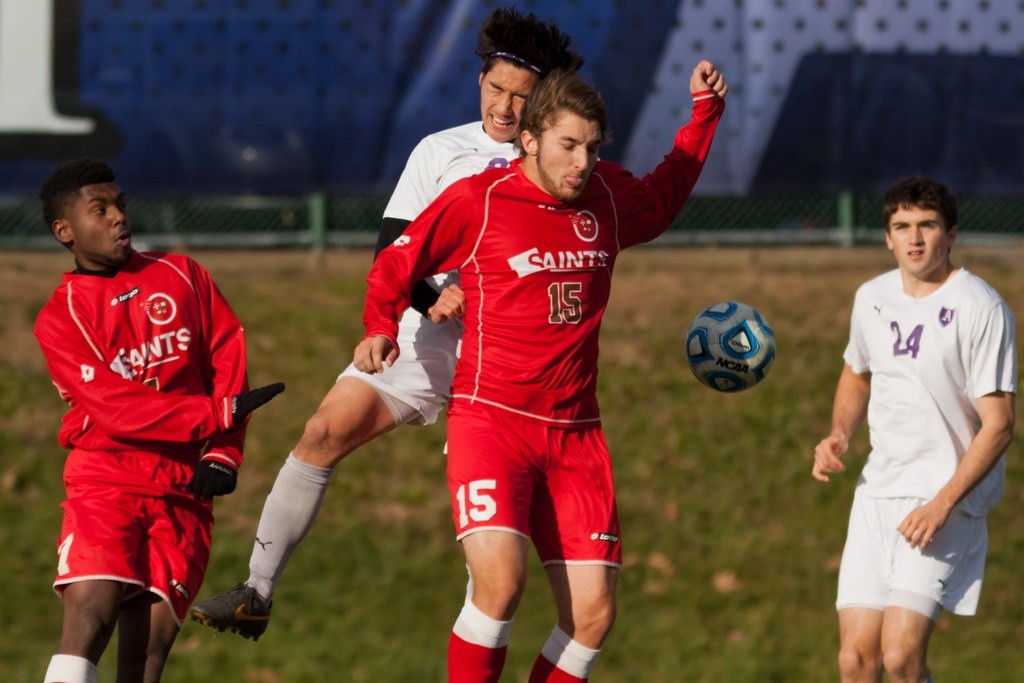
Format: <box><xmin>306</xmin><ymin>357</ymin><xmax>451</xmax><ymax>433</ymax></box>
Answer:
<box><xmin>189</xmin><ymin>582</ymin><xmax>273</xmax><ymax>642</ymax></box>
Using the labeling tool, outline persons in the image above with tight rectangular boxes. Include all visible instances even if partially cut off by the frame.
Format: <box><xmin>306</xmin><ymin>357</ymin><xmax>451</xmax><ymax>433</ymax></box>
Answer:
<box><xmin>353</xmin><ymin>59</ymin><xmax>729</xmax><ymax>683</ymax></box>
<box><xmin>812</xmin><ymin>176</ymin><xmax>1017</xmax><ymax>683</ymax></box>
<box><xmin>34</xmin><ymin>159</ymin><xmax>285</xmax><ymax>683</ymax></box>
<box><xmin>190</xmin><ymin>7</ymin><xmax>603</xmax><ymax>641</ymax></box>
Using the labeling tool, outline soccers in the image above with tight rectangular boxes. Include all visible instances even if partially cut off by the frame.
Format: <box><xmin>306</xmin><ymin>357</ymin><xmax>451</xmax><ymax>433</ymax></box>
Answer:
<box><xmin>686</xmin><ymin>300</ymin><xmax>777</xmax><ymax>394</ymax></box>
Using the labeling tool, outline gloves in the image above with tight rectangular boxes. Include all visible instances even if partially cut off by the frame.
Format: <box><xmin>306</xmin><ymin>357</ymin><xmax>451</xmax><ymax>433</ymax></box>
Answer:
<box><xmin>227</xmin><ymin>381</ymin><xmax>287</xmax><ymax>423</ymax></box>
<box><xmin>187</xmin><ymin>459</ymin><xmax>238</xmax><ymax>500</ymax></box>
